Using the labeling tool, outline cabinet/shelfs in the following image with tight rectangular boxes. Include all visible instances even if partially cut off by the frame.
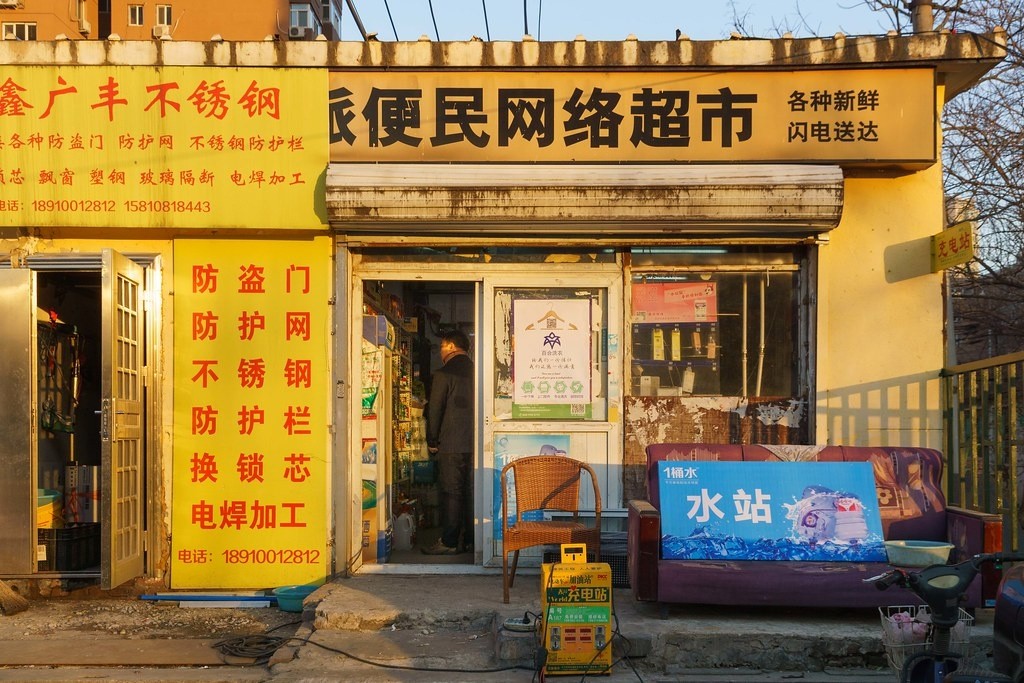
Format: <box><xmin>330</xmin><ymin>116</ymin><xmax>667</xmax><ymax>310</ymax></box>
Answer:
<box><xmin>364</xmin><ymin>293</ymin><xmax>428</xmax><ymax>533</ymax></box>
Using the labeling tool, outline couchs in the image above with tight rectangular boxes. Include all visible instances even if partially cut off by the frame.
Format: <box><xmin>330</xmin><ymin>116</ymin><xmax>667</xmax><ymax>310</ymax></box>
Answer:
<box><xmin>627</xmin><ymin>442</ymin><xmax>1003</xmax><ymax>621</ymax></box>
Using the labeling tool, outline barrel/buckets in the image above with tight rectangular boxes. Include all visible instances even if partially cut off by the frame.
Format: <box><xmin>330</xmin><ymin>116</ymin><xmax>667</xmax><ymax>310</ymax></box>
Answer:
<box><xmin>395</xmin><ymin>508</ymin><xmax>416</xmax><ymax>551</ymax></box>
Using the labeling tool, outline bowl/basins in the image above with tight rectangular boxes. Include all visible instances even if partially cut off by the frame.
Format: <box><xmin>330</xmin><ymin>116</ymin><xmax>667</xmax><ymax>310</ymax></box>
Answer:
<box><xmin>882</xmin><ymin>540</ymin><xmax>955</xmax><ymax>567</ymax></box>
<box><xmin>273</xmin><ymin>585</ymin><xmax>320</xmax><ymax>613</ymax></box>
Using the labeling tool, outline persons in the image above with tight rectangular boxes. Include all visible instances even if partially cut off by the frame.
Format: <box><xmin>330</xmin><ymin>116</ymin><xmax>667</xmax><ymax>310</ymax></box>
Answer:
<box><xmin>420</xmin><ymin>331</ymin><xmax>475</xmax><ymax>555</ymax></box>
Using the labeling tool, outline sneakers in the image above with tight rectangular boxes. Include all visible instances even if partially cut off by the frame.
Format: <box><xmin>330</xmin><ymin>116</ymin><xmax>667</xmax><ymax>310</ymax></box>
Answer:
<box><xmin>421</xmin><ymin>538</ymin><xmax>457</xmax><ymax>555</ymax></box>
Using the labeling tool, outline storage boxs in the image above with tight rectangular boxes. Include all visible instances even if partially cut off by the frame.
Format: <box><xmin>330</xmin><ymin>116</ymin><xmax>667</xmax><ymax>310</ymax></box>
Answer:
<box><xmin>38</xmin><ymin>522</ymin><xmax>100</xmax><ymax>572</ymax></box>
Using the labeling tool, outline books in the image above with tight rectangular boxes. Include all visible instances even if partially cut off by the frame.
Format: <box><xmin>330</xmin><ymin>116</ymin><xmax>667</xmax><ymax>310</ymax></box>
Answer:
<box><xmin>38</xmin><ymin>464</ymin><xmax>64</xmax><ymax>492</ymax></box>
<box><xmin>64</xmin><ymin>460</ymin><xmax>101</xmax><ymax>524</ymax></box>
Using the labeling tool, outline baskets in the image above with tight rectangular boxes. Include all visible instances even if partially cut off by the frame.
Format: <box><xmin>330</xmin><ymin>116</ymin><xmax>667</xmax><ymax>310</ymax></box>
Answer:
<box><xmin>879</xmin><ymin>604</ymin><xmax>974</xmax><ymax>678</ymax></box>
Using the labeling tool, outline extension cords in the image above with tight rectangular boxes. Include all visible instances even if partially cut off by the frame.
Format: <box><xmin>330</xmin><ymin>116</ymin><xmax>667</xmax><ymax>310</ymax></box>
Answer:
<box><xmin>504</xmin><ymin>617</ymin><xmax>540</xmax><ymax>632</ymax></box>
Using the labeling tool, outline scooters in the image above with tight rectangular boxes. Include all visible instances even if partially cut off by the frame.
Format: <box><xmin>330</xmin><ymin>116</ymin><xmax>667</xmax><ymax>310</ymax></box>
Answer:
<box><xmin>860</xmin><ymin>550</ymin><xmax>1023</xmax><ymax>683</ymax></box>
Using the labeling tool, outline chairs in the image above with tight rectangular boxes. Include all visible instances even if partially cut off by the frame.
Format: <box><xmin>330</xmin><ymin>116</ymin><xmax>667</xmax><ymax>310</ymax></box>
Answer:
<box><xmin>500</xmin><ymin>456</ymin><xmax>602</xmax><ymax>602</ymax></box>
